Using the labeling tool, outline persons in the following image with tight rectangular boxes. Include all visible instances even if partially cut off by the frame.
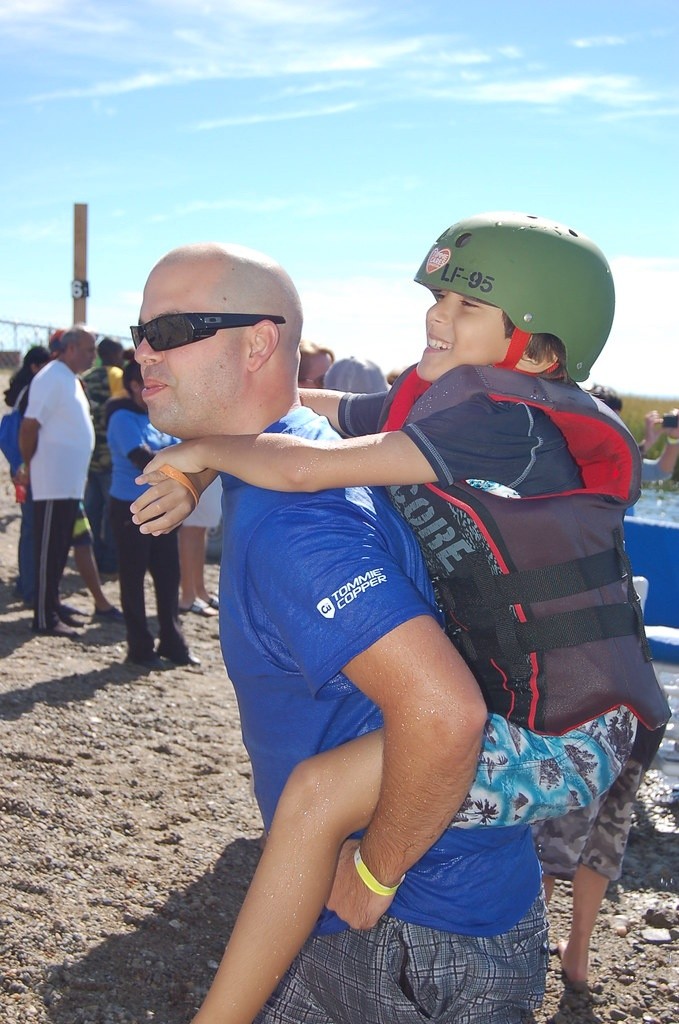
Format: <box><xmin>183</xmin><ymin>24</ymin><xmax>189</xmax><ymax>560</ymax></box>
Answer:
<box><xmin>136</xmin><ymin>244</ymin><xmax>552</xmax><ymax>1024</ymax></box>
<box><xmin>128</xmin><ymin>214</ymin><xmax>669</xmax><ymax>1024</ymax></box>
<box><xmin>4</xmin><ymin>329</ymin><xmax>679</xmax><ymax>670</ymax></box>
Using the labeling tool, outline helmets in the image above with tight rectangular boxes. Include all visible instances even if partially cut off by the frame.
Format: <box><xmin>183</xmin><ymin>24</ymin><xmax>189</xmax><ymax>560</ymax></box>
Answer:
<box><xmin>414</xmin><ymin>212</ymin><xmax>617</xmax><ymax>382</ymax></box>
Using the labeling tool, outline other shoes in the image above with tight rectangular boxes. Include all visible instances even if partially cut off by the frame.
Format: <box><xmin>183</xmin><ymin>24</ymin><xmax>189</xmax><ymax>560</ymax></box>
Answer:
<box><xmin>162</xmin><ymin>652</ymin><xmax>201</xmax><ymax>668</ymax></box>
<box><xmin>59</xmin><ymin>613</ymin><xmax>84</xmax><ymax>627</ymax></box>
<box><xmin>95</xmin><ymin>604</ymin><xmax>126</xmax><ymax>623</ymax></box>
<box><xmin>133</xmin><ymin>650</ymin><xmax>175</xmax><ymax>670</ymax></box>
<box><xmin>38</xmin><ymin>621</ymin><xmax>77</xmax><ymax>637</ymax></box>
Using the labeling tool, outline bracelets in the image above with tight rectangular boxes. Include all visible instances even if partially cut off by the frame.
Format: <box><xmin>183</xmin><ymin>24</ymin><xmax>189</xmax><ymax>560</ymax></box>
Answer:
<box><xmin>353</xmin><ymin>847</ymin><xmax>407</xmax><ymax>897</ymax></box>
<box><xmin>666</xmin><ymin>436</ymin><xmax>679</xmax><ymax>445</ymax></box>
<box><xmin>158</xmin><ymin>466</ymin><xmax>202</xmax><ymax>511</ymax></box>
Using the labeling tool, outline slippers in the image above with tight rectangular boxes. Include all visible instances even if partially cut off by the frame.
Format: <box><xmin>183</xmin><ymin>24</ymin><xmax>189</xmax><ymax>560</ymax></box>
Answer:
<box><xmin>180</xmin><ymin>596</ymin><xmax>213</xmax><ymax>617</ymax></box>
<box><xmin>206</xmin><ymin>595</ymin><xmax>220</xmax><ymax>609</ymax></box>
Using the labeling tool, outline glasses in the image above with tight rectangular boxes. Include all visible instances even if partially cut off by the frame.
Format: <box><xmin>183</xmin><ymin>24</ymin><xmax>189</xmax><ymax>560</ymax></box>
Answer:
<box><xmin>130</xmin><ymin>312</ymin><xmax>286</xmax><ymax>352</ymax></box>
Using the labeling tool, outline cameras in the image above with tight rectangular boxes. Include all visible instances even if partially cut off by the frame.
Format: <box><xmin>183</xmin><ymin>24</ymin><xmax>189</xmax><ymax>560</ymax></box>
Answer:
<box><xmin>662</xmin><ymin>414</ymin><xmax>679</xmax><ymax>428</ymax></box>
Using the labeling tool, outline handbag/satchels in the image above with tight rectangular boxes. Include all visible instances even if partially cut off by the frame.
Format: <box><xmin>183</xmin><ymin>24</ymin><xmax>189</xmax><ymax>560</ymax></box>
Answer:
<box><xmin>0</xmin><ymin>409</ymin><xmax>25</xmax><ymax>478</ymax></box>
